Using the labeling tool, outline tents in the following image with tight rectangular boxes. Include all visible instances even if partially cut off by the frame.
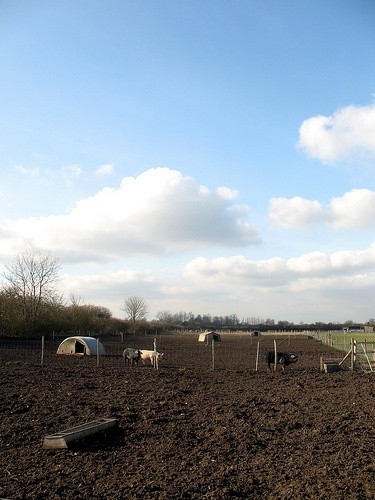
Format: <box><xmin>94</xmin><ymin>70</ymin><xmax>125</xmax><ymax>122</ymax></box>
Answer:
<box><xmin>197</xmin><ymin>331</ymin><xmax>221</xmax><ymax>343</ymax></box>
<box><xmin>55</xmin><ymin>336</ymin><xmax>105</xmax><ymax>356</ymax></box>
<box><xmin>250</xmin><ymin>330</ymin><xmax>261</xmax><ymax>337</ymax></box>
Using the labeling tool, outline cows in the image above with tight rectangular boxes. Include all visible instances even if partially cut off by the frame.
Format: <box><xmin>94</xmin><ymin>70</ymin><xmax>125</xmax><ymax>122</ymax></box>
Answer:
<box><xmin>123</xmin><ymin>348</ymin><xmax>144</xmax><ymax>367</ymax></box>
<box><xmin>264</xmin><ymin>351</ymin><xmax>299</xmax><ymax>371</ymax></box>
<box><xmin>138</xmin><ymin>349</ymin><xmax>164</xmax><ymax>370</ymax></box>
